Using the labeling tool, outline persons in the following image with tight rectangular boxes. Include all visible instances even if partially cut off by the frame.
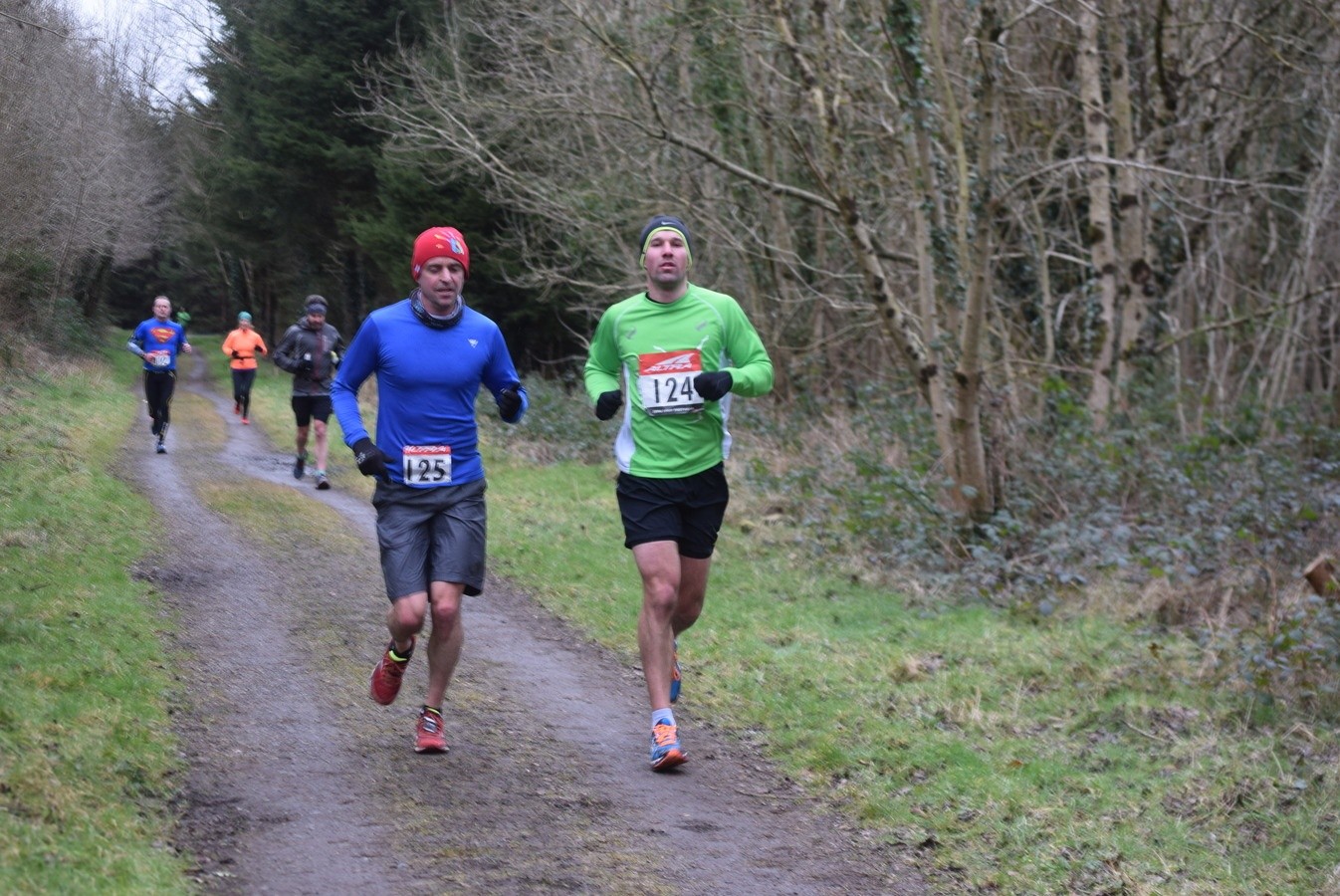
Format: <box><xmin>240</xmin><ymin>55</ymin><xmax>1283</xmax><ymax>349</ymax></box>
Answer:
<box><xmin>330</xmin><ymin>227</ymin><xmax>529</xmax><ymax>754</ymax></box>
<box><xmin>272</xmin><ymin>295</ymin><xmax>346</xmax><ymax>489</ymax></box>
<box><xmin>583</xmin><ymin>214</ymin><xmax>777</xmax><ymax>773</ymax></box>
<box><xmin>126</xmin><ymin>295</ymin><xmax>192</xmax><ymax>454</ymax></box>
<box><xmin>222</xmin><ymin>312</ymin><xmax>267</xmax><ymax>426</ymax></box>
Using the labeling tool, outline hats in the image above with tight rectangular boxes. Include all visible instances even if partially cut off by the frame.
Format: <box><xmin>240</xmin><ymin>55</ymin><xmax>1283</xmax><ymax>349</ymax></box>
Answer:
<box><xmin>410</xmin><ymin>227</ymin><xmax>470</xmax><ymax>280</ymax></box>
<box><xmin>638</xmin><ymin>214</ymin><xmax>693</xmax><ymax>272</ymax></box>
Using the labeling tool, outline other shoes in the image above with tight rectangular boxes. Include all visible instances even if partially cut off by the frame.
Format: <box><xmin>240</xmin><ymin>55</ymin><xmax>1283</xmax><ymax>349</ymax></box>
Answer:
<box><xmin>157</xmin><ymin>445</ymin><xmax>165</xmax><ymax>452</ymax></box>
<box><xmin>242</xmin><ymin>417</ymin><xmax>249</xmax><ymax>426</ymax></box>
<box><xmin>294</xmin><ymin>458</ymin><xmax>305</xmax><ymax>478</ymax></box>
<box><xmin>316</xmin><ymin>474</ymin><xmax>329</xmax><ymax>490</ymax></box>
<box><xmin>152</xmin><ymin>419</ymin><xmax>157</xmax><ymax>435</ymax></box>
<box><xmin>235</xmin><ymin>403</ymin><xmax>240</xmax><ymax>414</ymax></box>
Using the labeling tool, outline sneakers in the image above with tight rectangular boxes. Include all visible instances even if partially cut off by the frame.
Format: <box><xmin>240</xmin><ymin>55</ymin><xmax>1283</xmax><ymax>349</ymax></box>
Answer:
<box><xmin>413</xmin><ymin>707</ymin><xmax>450</xmax><ymax>752</ymax></box>
<box><xmin>649</xmin><ymin>719</ymin><xmax>689</xmax><ymax>770</ymax></box>
<box><xmin>371</xmin><ymin>637</ymin><xmax>417</xmax><ymax>705</ymax></box>
<box><xmin>668</xmin><ymin>638</ymin><xmax>681</xmax><ymax>701</ymax></box>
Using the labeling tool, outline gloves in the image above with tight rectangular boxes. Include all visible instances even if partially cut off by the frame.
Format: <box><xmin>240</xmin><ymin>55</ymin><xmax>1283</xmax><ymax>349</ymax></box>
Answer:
<box><xmin>353</xmin><ymin>440</ymin><xmax>395</xmax><ymax>483</ymax></box>
<box><xmin>255</xmin><ymin>345</ymin><xmax>262</xmax><ymax>351</ymax></box>
<box><xmin>693</xmin><ymin>371</ymin><xmax>733</xmax><ymax>403</ymax></box>
<box><xmin>596</xmin><ymin>390</ymin><xmax>623</xmax><ymax>420</ymax></box>
<box><xmin>498</xmin><ymin>382</ymin><xmax>523</xmax><ymax>422</ymax></box>
<box><xmin>299</xmin><ymin>360</ymin><xmax>312</xmax><ymax>372</ymax></box>
<box><xmin>233</xmin><ymin>351</ymin><xmax>237</xmax><ymax>357</ymax></box>
